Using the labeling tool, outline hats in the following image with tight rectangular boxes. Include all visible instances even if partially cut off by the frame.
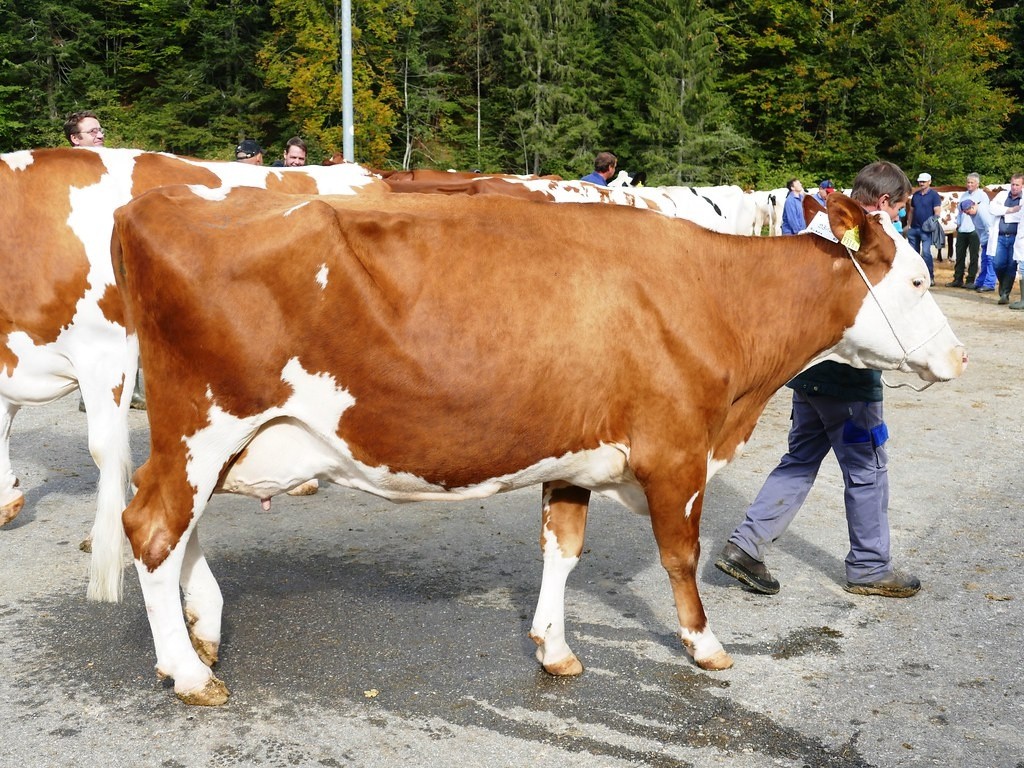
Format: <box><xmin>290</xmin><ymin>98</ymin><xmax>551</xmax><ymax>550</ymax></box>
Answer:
<box><xmin>917</xmin><ymin>173</ymin><xmax>931</xmax><ymax>181</ymax></box>
<box><xmin>820</xmin><ymin>181</ymin><xmax>834</xmax><ymax>193</ymax></box>
<box><xmin>960</xmin><ymin>199</ymin><xmax>974</xmax><ymax>211</ymax></box>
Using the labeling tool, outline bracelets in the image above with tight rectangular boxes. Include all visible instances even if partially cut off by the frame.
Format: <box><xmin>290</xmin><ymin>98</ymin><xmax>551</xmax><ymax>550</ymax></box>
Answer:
<box><xmin>909</xmin><ymin>227</ymin><xmax>911</xmax><ymax>229</ymax></box>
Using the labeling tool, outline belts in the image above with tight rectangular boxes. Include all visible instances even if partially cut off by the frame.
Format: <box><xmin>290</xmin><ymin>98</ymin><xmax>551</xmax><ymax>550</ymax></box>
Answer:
<box><xmin>999</xmin><ymin>232</ymin><xmax>1016</xmax><ymax>237</ymax></box>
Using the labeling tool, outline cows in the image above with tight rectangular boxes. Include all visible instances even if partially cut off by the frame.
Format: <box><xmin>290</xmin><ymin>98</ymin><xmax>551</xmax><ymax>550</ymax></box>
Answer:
<box><xmin>1</xmin><ymin>146</ymin><xmax>967</xmax><ymax>707</ymax></box>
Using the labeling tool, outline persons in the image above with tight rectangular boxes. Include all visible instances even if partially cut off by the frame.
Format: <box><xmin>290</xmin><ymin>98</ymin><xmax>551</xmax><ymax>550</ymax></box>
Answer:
<box><xmin>813</xmin><ymin>180</ymin><xmax>833</xmax><ymax>207</ymax></box>
<box><xmin>945</xmin><ymin>172</ymin><xmax>989</xmax><ymax>288</ymax></box>
<box><xmin>63</xmin><ymin>113</ymin><xmax>147</xmax><ymax>411</ymax></box>
<box><xmin>989</xmin><ymin>173</ymin><xmax>1024</xmax><ymax>310</ymax></box>
<box><xmin>234</xmin><ymin>138</ymin><xmax>304</xmax><ymax>168</ymax></box>
<box><xmin>959</xmin><ymin>200</ymin><xmax>996</xmax><ymax>291</ymax></box>
<box><xmin>580</xmin><ymin>151</ymin><xmax>617</xmax><ymax>186</ymax></box>
<box><xmin>781</xmin><ymin>178</ymin><xmax>805</xmax><ymax>235</ymax></box>
<box><xmin>715</xmin><ymin>161</ymin><xmax>920</xmax><ymax>599</ymax></box>
<box><xmin>893</xmin><ymin>173</ymin><xmax>941</xmax><ymax>286</ymax></box>
<box><xmin>938</xmin><ymin>234</ymin><xmax>955</xmax><ymax>261</ymax></box>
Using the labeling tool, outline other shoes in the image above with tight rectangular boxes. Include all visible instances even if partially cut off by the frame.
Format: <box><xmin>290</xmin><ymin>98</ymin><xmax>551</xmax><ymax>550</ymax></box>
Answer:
<box><xmin>977</xmin><ymin>286</ymin><xmax>995</xmax><ymax>292</ymax></box>
<box><xmin>961</xmin><ymin>282</ymin><xmax>973</xmax><ymax>288</ymax></box>
<box><xmin>945</xmin><ymin>280</ymin><xmax>963</xmax><ymax>287</ymax></box>
<box><xmin>930</xmin><ymin>278</ymin><xmax>935</xmax><ymax>286</ymax></box>
<box><xmin>970</xmin><ymin>284</ymin><xmax>982</xmax><ymax>289</ymax></box>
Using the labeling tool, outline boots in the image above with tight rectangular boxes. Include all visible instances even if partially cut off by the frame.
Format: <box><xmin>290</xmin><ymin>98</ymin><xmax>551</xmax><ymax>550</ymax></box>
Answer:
<box><xmin>998</xmin><ymin>275</ymin><xmax>1015</xmax><ymax>304</ymax></box>
<box><xmin>1009</xmin><ymin>280</ymin><xmax>1024</xmax><ymax>309</ymax></box>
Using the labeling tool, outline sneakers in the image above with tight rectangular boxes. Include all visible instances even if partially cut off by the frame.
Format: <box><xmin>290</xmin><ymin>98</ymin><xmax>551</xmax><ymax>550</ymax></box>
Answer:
<box><xmin>844</xmin><ymin>567</ymin><xmax>921</xmax><ymax>598</ymax></box>
<box><xmin>715</xmin><ymin>542</ymin><xmax>780</xmax><ymax>594</ymax></box>
